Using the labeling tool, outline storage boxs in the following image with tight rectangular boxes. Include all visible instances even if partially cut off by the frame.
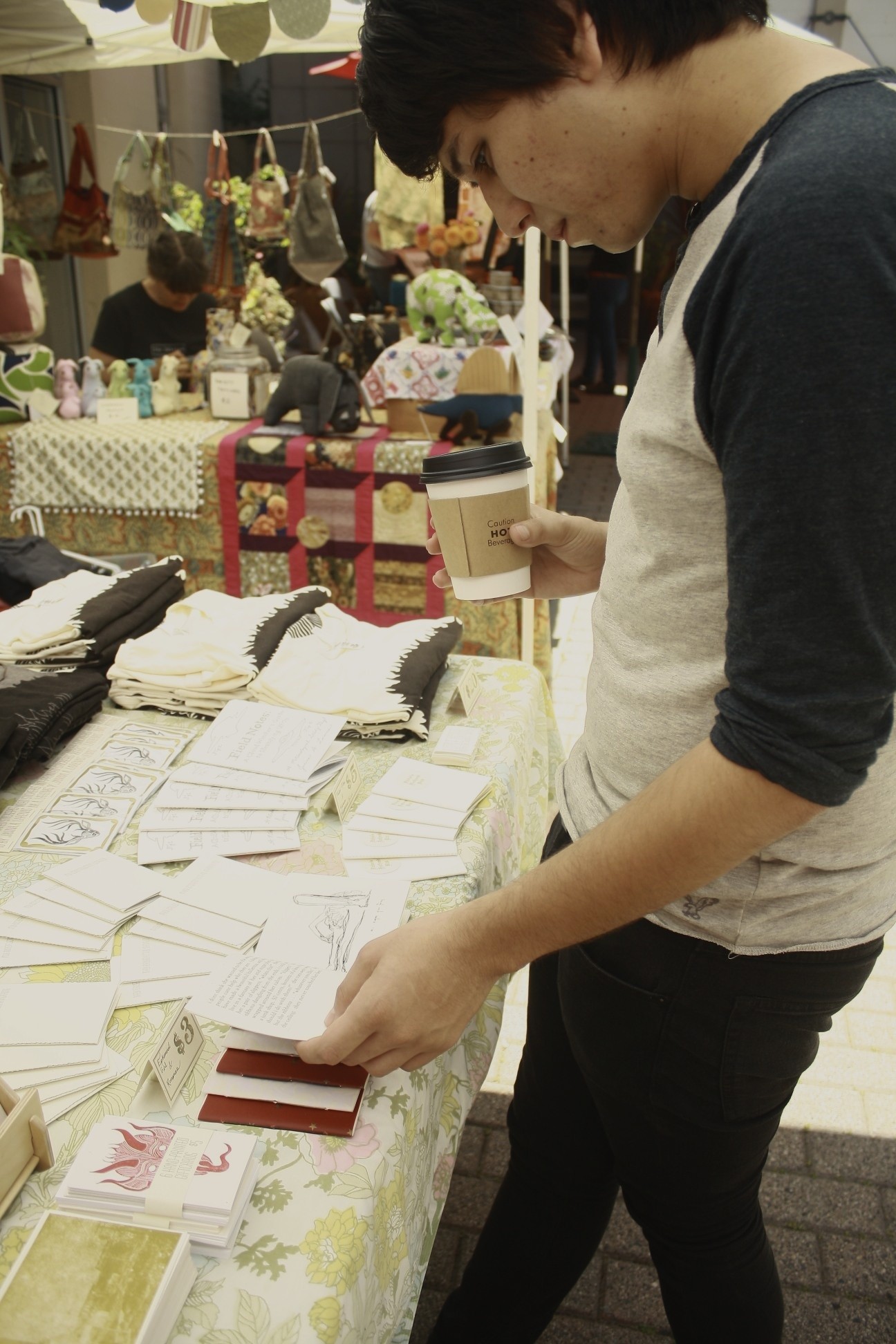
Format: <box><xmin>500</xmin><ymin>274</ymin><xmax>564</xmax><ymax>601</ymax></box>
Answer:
<box><xmin>387</xmin><ymin>342</ymin><xmax>515</xmax><ymax>436</ymax></box>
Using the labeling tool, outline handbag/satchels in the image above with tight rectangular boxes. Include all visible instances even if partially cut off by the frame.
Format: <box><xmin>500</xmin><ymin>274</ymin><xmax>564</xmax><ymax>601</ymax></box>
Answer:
<box><xmin>2</xmin><ymin>102</ymin><xmax>508</xmax><ymax>285</ymax></box>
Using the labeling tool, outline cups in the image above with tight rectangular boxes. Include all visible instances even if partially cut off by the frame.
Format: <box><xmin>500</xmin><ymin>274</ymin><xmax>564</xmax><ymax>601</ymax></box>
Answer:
<box><xmin>420</xmin><ymin>440</ymin><xmax>534</xmax><ymax>600</ymax></box>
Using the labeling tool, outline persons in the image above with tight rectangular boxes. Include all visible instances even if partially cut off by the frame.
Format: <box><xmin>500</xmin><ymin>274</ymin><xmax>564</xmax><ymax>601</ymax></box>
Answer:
<box><xmin>570</xmin><ymin>238</ymin><xmax>634</xmax><ymax>397</ymax></box>
<box><xmin>356</xmin><ymin>2</ymin><xmax>896</xmax><ymax>1344</ymax></box>
<box><xmin>89</xmin><ymin>228</ymin><xmax>218</xmax><ymax>391</ymax></box>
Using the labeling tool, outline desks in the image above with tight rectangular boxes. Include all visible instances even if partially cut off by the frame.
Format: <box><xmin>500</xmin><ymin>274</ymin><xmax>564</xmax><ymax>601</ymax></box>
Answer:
<box><xmin>0</xmin><ymin>654</ymin><xmax>568</xmax><ymax>1344</ymax></box>
<box><xmin>0</xmin><ymin>405</ymin><xmax>559</xmax><ymax>678</ymax></box>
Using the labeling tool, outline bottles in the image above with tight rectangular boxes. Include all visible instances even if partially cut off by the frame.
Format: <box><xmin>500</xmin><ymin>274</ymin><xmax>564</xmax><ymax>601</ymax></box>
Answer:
<box><xmin>205</xmin><ymin>345</ymin><xmax>271</xmax><ymax>421</ymax></box>
